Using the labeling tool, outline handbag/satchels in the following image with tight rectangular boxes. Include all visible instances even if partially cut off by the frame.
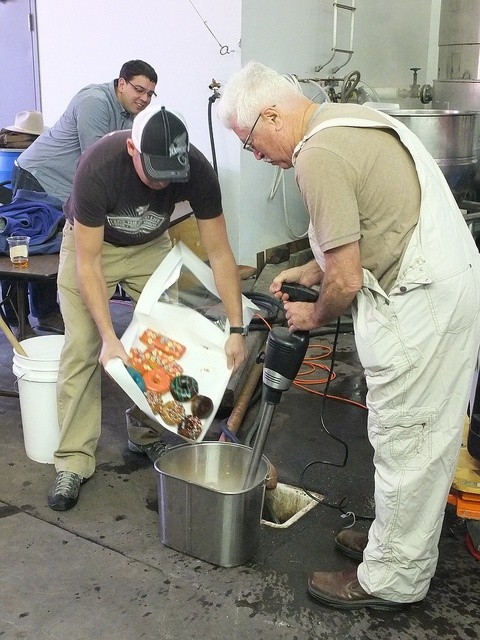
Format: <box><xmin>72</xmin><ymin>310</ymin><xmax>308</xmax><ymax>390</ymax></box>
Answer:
<box><xmin>0</xmin><ymin>131</ymin><xmax>39</xmax><ymax>149</ymax></box>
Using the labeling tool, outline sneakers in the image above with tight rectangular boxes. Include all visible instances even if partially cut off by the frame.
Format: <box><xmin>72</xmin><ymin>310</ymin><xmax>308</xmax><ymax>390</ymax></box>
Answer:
<box><xmin>48</xmin><ymin>471</ymin><xmax>83</xmax><ymax>511</ymax></box>
<box><xmin>128</xmin><ymin>439</ymin><xmax>166</xmax><ymax>462</ymax></box>
<box><xmin>37</xmin><ymin>312</ymin><xmax>65</xmax><ymax>335</ymax></box>
<box><xmin>9</xmin><ymin>322</ymin><xmax>38</xmax><ymax>342</ymax></box>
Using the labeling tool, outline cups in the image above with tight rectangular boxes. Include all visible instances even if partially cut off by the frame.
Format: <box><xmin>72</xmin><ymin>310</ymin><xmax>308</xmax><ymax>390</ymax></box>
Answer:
<box><xmin>7</xmin><ymin>236</ymin><xmax>31</xmax><ymax>269</ymax></box>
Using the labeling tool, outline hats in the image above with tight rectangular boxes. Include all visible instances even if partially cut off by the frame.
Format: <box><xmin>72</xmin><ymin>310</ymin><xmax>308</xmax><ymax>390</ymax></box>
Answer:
<box><xmin>0</xmin><ymin>111</ymin><xmax>51</xmax><ymax>135</ymax></box>
<box><xmin>132</xmin><ymin>106</ymin><xmax>191</xmax><ymax>183</ymax></box>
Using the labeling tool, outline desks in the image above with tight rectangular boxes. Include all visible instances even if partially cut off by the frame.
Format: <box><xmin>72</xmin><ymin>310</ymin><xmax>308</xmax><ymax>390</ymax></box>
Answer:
<box><xmin>1</xmin><ymin>255</ymin><xmax>59</xmax><ymax>342</ymax></box>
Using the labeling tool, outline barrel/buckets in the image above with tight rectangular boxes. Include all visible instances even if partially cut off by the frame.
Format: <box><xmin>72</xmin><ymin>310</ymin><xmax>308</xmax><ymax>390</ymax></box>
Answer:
<box><xmin>153</xmin><ymin>440</ymin><xmax>271</xmax><ymax>569</ymax></box>
<box><xmin>12</xmin><ymin>335</ymin><xmax>63</xmax><ymax>464</ymax></box>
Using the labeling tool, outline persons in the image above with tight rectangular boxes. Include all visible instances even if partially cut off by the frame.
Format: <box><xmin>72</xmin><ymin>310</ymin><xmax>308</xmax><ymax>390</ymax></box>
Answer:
<box><xmin>219</xmin><ymin>63</ymin><xmax>480</xmax><ymax>613</ymax></box>
<box><xmin>13</xmin><ymin>60</ymin><xmax>157</xmax><ymax>203</ymax></box>
<box><xmin>48</xmin><ymin>106</ymin><xmax>248</xmax><ymax>511</ymax></box>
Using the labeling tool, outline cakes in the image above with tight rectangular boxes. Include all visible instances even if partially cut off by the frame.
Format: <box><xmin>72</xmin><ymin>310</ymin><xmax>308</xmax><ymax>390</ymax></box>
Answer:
<box><xmin>130</xmin><ymin>346</ymin><xmax>150</xmax><ymax>372</ymax></box>
<box><xmin>140</xmin><ymin>329</ymin><xmax>186</xmax><ymax>360</ymax></box>
<box><xmin>143</xmin><ymin>345</ymin><xmax>184</xmax><ymax>379</ymax></box>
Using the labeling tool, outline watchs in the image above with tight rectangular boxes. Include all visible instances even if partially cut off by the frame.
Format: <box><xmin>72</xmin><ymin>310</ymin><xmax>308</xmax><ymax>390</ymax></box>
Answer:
<box><xmin>230</xmin><ymin>327</ymin><xmax>249</xmax><ymax>336</ymax></box>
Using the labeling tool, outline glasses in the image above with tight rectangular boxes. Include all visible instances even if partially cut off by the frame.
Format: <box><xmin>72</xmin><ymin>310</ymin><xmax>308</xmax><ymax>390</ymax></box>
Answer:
<box><xmin>125</xmin><ymin>79</ymin><xmax>157</xmax><ymax>99</ymax></box>
<box><xmin>242</xmin><ymin>105</ymin><xmax>276</xmax><ymax>152</ymax></box>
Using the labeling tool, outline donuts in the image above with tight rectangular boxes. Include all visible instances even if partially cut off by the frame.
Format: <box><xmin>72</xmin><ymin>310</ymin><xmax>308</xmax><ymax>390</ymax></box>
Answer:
<box><xmin>160</xmin><ymin>401</ymin><xmax>184</xmax><ymax>424</ymax></box>
<box><xmin>127</xmin><ymin>366</ymin><xmax>145</xmax><ymax>391</ymax></box>
<box><xmin>143</xmin><ymin>369</ymin><xmax>170</xmax><ymax>394</ymax></box>
<box><xmin>170</xmin><ymin>375</ymin><xmax>198</xmax><ymax>401</ymax></box>
<box><xmin>178</xmin><ymin>414</ymin><xmax>202</xmax><ymax>441</ymax></box>
<box><xmin>144</xmin><ymin>388</ymin><xmax>162</xmax><ymax>415</ymax></box>
<box><xmin>192</xmin><ymin>395</ymin><xmax>213</xmax><ymax>419</ymax></box>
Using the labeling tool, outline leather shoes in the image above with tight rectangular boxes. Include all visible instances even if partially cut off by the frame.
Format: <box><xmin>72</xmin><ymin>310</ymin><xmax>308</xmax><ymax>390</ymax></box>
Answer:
<box><xmin>307</xmin><ymin>569</ymin><xmax>409</xmax><ymax>611</ymax></box>
<box><xmin>334</xmin><ymin>530</ymin><xmax>368</xmax><ymax>561</ymax></box>
